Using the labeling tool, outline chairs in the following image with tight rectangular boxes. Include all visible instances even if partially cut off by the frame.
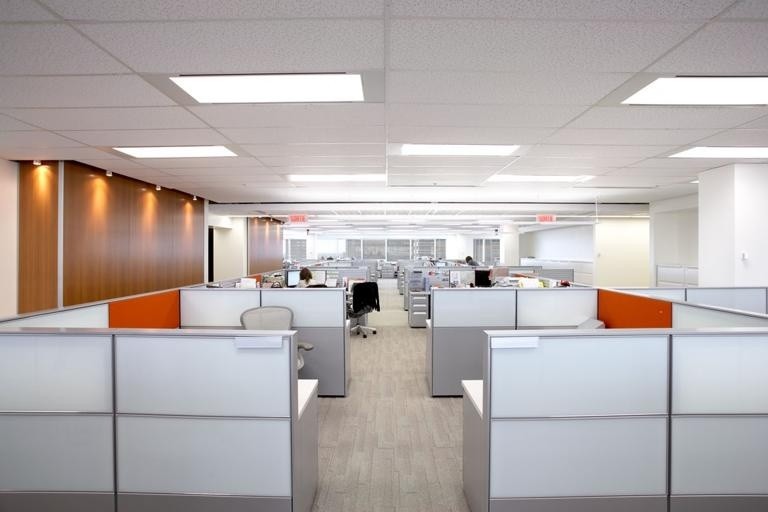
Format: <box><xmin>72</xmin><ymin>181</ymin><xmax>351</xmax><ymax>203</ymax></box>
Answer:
<box><xmin>238</xmin><ymin>301</ymin><xmax>316</xmax><ymax>373</ymax></box>
<box><xmin>346</xmin><ymin>280</ymin><xmax>382</xmax><ymax>341</ymax></box>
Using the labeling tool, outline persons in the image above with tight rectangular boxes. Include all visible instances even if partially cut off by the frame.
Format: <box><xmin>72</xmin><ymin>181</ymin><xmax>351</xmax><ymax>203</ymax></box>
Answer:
<box><xmin>466</xmin><ymin>256</ymin><xmax>480</xmax><ymax>266</ymax></box>
<box><xmin>294</xmin><ymin>269</ymin><xmax>317</xmax><ymax>288</ymax></box>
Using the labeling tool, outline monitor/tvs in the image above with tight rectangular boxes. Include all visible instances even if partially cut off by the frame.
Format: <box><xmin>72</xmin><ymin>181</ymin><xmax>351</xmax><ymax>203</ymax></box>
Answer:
<box><xmin>449</xmin><ymin>270</ymin><xmax>476</xmax><ymax>288</ymax></box>
<box><xmin>285</xmin><ymin>269</ymin><xmax>303</xmax><ymax>287</ymax></box>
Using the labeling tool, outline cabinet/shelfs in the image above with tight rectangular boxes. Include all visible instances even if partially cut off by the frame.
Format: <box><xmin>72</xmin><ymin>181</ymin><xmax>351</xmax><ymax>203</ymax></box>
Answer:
<box><xmin>409</xmin><ymin>294</ymin><xmax>429</xmax><ymax>329</ymax></box>
